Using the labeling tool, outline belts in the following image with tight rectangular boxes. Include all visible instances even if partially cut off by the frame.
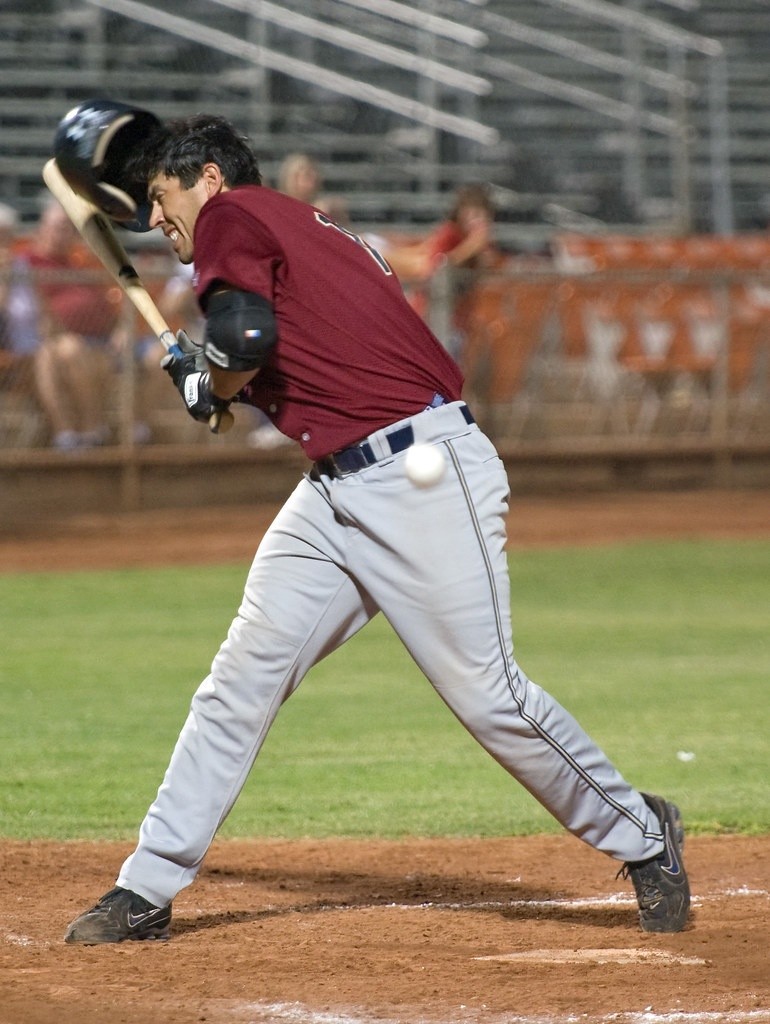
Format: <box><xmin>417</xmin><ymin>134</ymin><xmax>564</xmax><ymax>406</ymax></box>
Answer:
<box><xmin>310</xmin><ymin>405</ymin><xmax>476</xmax><ymax>481</ymax></box>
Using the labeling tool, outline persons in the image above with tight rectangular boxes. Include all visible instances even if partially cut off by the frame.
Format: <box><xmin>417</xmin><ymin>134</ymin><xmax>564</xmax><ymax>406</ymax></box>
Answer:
<box><xmin>0</xmin><ymin>149</ymin><xmax>502</xmax><ymax>449</ymax></box>
<box><xmin>66</xmin><ymin>110</ymin><xmax>691</xmax><ymax>943</ymax></box>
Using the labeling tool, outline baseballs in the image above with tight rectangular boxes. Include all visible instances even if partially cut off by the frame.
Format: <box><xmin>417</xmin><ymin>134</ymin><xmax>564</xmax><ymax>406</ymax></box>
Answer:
<box><xmin>405</xmin><ymin>444</ymin><xmax>447</xmax><ymax>488</ymax></box>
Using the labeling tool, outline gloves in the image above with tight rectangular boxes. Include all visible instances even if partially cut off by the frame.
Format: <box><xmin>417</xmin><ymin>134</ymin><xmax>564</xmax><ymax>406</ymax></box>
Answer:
<box><xmin>168</xmin><ymin>354</ymin><xmax>232</xmax><ymax>422</ymax></box>
<box><xmin>161</xmin><ymin>328</ymin><xmax>240</xmax><ymax>403</ymax></box>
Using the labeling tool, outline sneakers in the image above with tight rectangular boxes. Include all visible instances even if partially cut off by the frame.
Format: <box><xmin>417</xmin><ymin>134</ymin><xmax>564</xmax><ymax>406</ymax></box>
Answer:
<box><xmin>64</xmin><ymin>887</ymin><xmax>172</xmax><ymax>946</ymax></box>
<box><xmin>615</xmin><ymin>792</ymin><xmax>690</xmax><ymax>933</ymax></box>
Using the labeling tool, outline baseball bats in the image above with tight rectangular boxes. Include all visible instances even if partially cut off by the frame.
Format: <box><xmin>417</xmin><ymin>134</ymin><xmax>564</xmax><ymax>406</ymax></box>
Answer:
<box><xmin>42</xmin><ymin>157</ymin><xmax>236</xmax><ymax>435</ymax></box>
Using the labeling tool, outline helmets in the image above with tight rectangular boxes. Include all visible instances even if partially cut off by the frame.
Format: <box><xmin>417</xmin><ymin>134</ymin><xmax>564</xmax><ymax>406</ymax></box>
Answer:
<box><xmin>52</xmin><ymin>100</ymin><xmax>162</xmax><ymax>234</ymax></box>
